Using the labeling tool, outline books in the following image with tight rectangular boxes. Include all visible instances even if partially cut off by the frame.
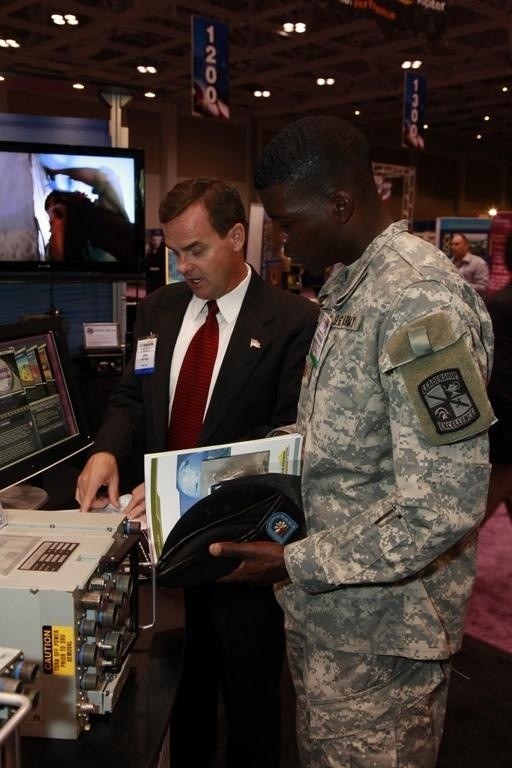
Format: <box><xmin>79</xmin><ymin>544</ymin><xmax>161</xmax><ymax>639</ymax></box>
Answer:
<box><xmin>0</xmin><ymin>318</ymin><xmax>93</xmax><ymax>489</ymax></box>
<box><xmin>135</xmin><ymin>433</ymin><xmax>311</xmax><ymax>559</ymax></box>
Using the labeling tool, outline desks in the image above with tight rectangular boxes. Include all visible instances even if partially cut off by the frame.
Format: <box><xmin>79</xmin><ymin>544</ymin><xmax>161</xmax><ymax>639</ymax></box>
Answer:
<box><xmin>0</xmin><ymin>444</ymin><xmax>191</xmax><ymax>767</ymax></box>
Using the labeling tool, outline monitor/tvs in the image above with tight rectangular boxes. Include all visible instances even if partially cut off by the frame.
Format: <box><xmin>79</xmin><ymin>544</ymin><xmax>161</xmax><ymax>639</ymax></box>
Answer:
<box><xmin>0</xmin><ymin>316</ymin><xmax>94</xmax><ymax>509</ymax></box>
<box><xmin>0</xmin><ymin>140</ymin><xmax>146</xmax><ymax>272</ymax></box>
<box><xmin>246</xmin><ymin>203</ymin><xmax>277</xmax><ymax>288</ymax></box>
<box><xmin>164</xmin><ymin>243</ymin><xmax>184</xmax><ymax>284</ymax></box>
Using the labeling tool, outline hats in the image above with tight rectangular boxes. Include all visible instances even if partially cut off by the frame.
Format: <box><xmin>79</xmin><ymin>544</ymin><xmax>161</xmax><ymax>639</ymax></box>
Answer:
<box><xmin>156</xmin><ymin>473</ymin><xmax>303</xmax><ymax>585</ymax></box>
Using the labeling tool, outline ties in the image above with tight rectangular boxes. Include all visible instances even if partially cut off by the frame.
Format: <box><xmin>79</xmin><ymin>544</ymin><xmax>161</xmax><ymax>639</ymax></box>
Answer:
<box><xmin>169</xmin><ymin>301</ymin><xmax>219</xmax><ymax>451</ymax></box>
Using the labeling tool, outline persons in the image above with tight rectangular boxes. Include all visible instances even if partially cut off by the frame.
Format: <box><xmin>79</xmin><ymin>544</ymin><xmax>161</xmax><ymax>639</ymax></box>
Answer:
<box><xmin>207</xmin><ymin>116</ymin><xmax>498</xmax><ymax>762</ymax></box>
<box><xmin>75</xmin><ymin>176</ymin><xmax>319</xmax><ymax>515</ymax></box>
<box><xmin>481</xmin><ymin>231</ymin><xmax>512</xmax><ymax>527</ymax></box>
<box><xmin>45</xmin><ymin>167</ymin><xmax>129</xmax><ymax>263</ymax></box>
<box><xmin>433</xmin><ymin>216</ymin><xmax>492</xmax><ymax>291</ymax></box>
